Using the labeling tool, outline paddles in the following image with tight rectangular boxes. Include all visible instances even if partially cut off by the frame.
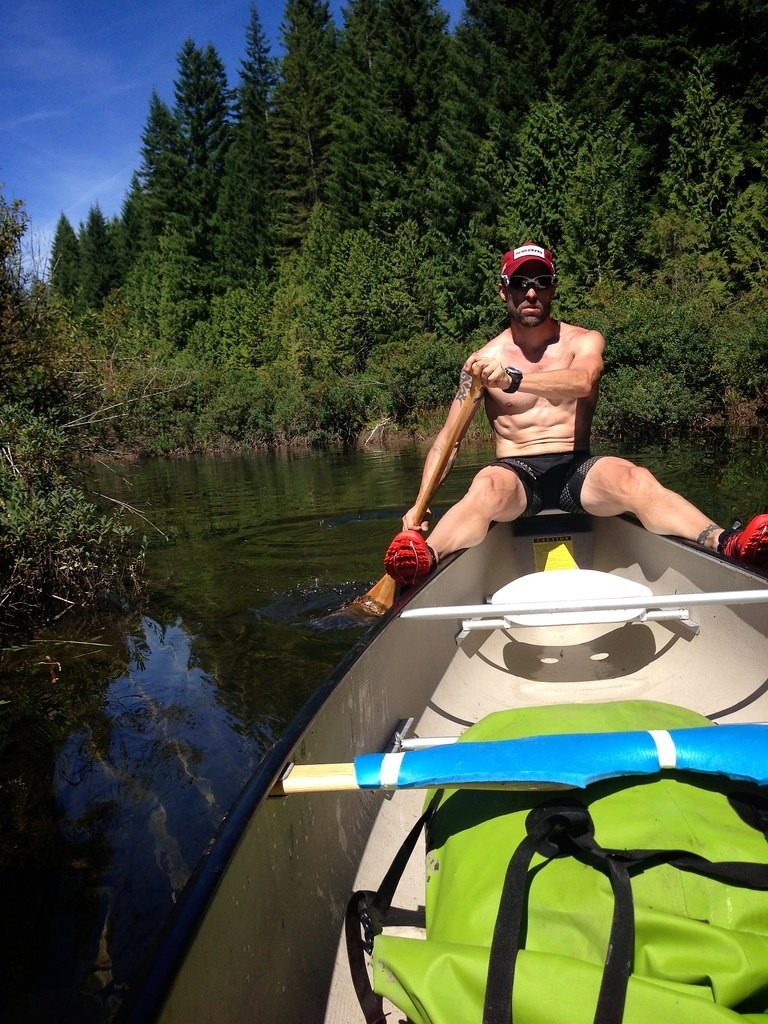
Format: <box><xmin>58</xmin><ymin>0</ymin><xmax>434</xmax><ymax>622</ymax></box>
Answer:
<box><xmin>339</xmin><ymin>356</ymin><xmax>487</xmax><ymax>618</ymax></box>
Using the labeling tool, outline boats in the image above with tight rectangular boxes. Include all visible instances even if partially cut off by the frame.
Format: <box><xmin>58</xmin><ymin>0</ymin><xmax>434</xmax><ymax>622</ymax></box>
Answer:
<box><xmin>124</xmin><ymin>507</ymin><xmax>768</xmax><ymax>1024</ymax></box>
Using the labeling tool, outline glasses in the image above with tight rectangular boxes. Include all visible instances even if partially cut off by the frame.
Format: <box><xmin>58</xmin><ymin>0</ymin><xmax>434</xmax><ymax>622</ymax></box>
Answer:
<box><xmin>503</xmin><ymin>275</ymin><xmax>558</xmax><ymax>292</ymax></box>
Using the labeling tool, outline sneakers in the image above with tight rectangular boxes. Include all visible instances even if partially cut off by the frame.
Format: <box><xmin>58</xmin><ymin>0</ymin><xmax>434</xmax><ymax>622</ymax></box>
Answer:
<box><xmin>384</xmin><ymin>530</ymin><xmax>437</xmax><ymax>607</ymax></box>
<box><xmin>717</xmin><ymin>514</ymin><xmax>768</xmax><ymax>561</ymax></box>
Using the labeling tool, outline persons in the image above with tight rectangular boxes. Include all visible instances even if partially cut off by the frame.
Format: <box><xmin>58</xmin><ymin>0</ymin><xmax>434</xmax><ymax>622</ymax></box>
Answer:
<box><xmin>384</xmin><ymin>241</ymin><xmax>768</xmax><ymax>589</ymax></box>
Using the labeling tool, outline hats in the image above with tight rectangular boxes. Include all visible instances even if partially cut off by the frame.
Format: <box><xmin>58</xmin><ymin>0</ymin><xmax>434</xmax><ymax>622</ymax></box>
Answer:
<box><xmin>501</xmin><ymin>242</ymin><xmax>555</xmax><ymax>279</ymax></box>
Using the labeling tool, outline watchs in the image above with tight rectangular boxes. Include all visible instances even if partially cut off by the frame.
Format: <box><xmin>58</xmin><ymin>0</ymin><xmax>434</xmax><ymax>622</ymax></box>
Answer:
<box><xmin>502</xmin><ymin>367</ymin><xmax>523</xmax><ymax>393</ymax></box>
